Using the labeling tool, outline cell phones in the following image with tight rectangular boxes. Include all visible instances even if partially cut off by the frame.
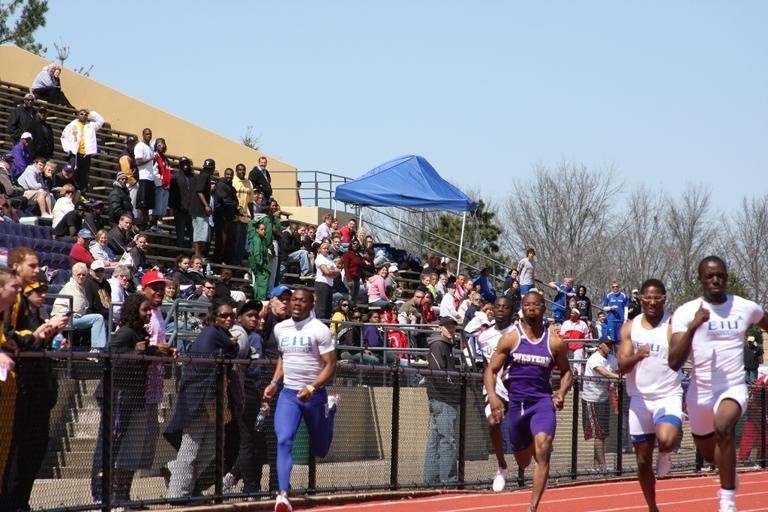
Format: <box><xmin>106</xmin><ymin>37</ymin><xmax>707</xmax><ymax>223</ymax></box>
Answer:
<box><xmin>65</xmin><ymin>311</ymin><xmax>73</xmax><ymax>318</ymax></box>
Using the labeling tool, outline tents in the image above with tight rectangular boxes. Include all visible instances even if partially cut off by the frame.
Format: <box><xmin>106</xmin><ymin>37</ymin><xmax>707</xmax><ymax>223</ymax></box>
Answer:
<box><xmin>334</xmin><ymin>155</ymin><xmax>475</xmax><ymax>275</ymax></box>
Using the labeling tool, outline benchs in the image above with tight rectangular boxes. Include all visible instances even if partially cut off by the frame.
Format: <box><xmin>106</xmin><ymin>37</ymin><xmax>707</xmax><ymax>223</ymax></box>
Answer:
<box><xmin>0</xmin><ymin>80</ymin><xmax>422</xmax><ymax>320</ymax></box>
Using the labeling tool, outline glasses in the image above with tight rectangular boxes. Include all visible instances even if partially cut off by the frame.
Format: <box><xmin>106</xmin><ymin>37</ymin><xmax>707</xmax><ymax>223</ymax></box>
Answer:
<box><xmin>352</xmin><ymin>316</ymin><xmax>361</xmax><ymax>319</ymax></box>
<box><xmin>218</xmin><ymin>312</ymin><xmax>236</xmax><ymax>318</ymax></box>
<box><xmin>341</xmin><ymin>304</ymin><xmax>350</xmax><ymax>307</ymax></box>
<box><xmin>642</xmin><ymin>295</ymin><xmax>665</xmax><ymax>302</ymax></box>
<box><xmin>205</xmin><ymin>287</ymin><xmax>216</xmax><ymax>290</ymax></box>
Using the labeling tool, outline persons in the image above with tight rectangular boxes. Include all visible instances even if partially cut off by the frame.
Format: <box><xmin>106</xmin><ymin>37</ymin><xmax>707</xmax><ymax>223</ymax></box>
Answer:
<box><xmin>485</xmin><ymin>291</ymin><xmax>573</xmax><ymax>512</ymax></box>
<box><xmin>617</xmin><ymin>278</ymin><xmax>683</xmax><ymax>512</ymax></box>
<box><xmin>1</xmin><ymin>61</ymin><xmax>408</xmax><ymax>512</ymax></box>
<box><xmin>399</xmin><ymin>249</ymin><xmax>763</xmax><ymax>491</ymax></box>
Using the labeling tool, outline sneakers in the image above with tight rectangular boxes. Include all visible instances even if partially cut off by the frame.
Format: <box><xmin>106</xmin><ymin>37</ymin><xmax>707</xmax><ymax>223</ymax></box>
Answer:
<box><xmin>656</xmin><ymin>451</ymin><xmax>672</xmax><ymax>477</ymax></box>
<box><xmin>493</xmin><ymin>466</ymin><xmax>510</xmax><ymax>492</ymax></box>
<box><xmin>274</xmin><ymin>496</ymin><xmax>293</xmax><ymax>512</ymax></box>
<box><xmin>326</xmin><ymin>394</ymin><xmax>342</xmax><ymax>408</ymax></box>
<box><xmin>719</xmin><ymin>490</ymin><xmax>736</xmax><ymax>512</ymax></box>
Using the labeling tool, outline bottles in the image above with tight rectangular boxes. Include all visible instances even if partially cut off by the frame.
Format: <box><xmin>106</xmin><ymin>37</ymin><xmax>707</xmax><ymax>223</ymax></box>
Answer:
<box><xmin>50</xmin><ymin>329</ymin><xmax>65</xmax><ymax>361</ymax></box>
<box><xmin>206</xmin><ymin>263</ymin><xmax>211</xmax><ymax>275</ymax></box>
<box><xmin>255</xmin><ymin>408</ymin><xmax>268</xmax><ymax>431</ymax></box>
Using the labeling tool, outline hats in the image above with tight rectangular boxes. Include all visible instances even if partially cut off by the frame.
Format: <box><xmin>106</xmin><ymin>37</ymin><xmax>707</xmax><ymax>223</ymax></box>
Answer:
<box><xmin>91</xmin><ymin>260</ymin><xmax>106</xmax><ymax>271</ymax></box>
<box><xmin>388</xmin><ymin>265</ymin><xmax>398</xmax><ymax>273</ymax></box>
<box><xmin>24</xmin><ymin>279</ymin><xmax>48</xmax><ymax>292</ymax></box>
<box><xmin>439</xmin><ymin>316</ymin><xmax>457</xmax><ymax>326</ymax></box>
<box><xmin>77</xmin><ymin>228</ymin><xmax>92</xmax><ymax>239</ymax></box>
<box><xmin>140</xmin><ymin>271</ymin><xmax>172</xmax><ymax>288</ymax></box>
<box><xmin>632</xmin><ymin>289</ymin><xmax>637</xmax><ymax>293</ymax></box>
<box><xmin>746</xmin><ymin>336</ymin><xmax>755</xmax><ymax>342</ymax></box>
<box><xmin>236</xmin><ymin>300</ymin><xmax>263</xmax><ymax>316</ymax></box>
<box><xmin>597</xmin><ymin>335</ymin><xmax>612</xmax><ymax>345</ymax></box>
<box><xmin>271</xmin><ymin>284</ymin><xmax>292</xmax><ymax>297</ymax></box>
